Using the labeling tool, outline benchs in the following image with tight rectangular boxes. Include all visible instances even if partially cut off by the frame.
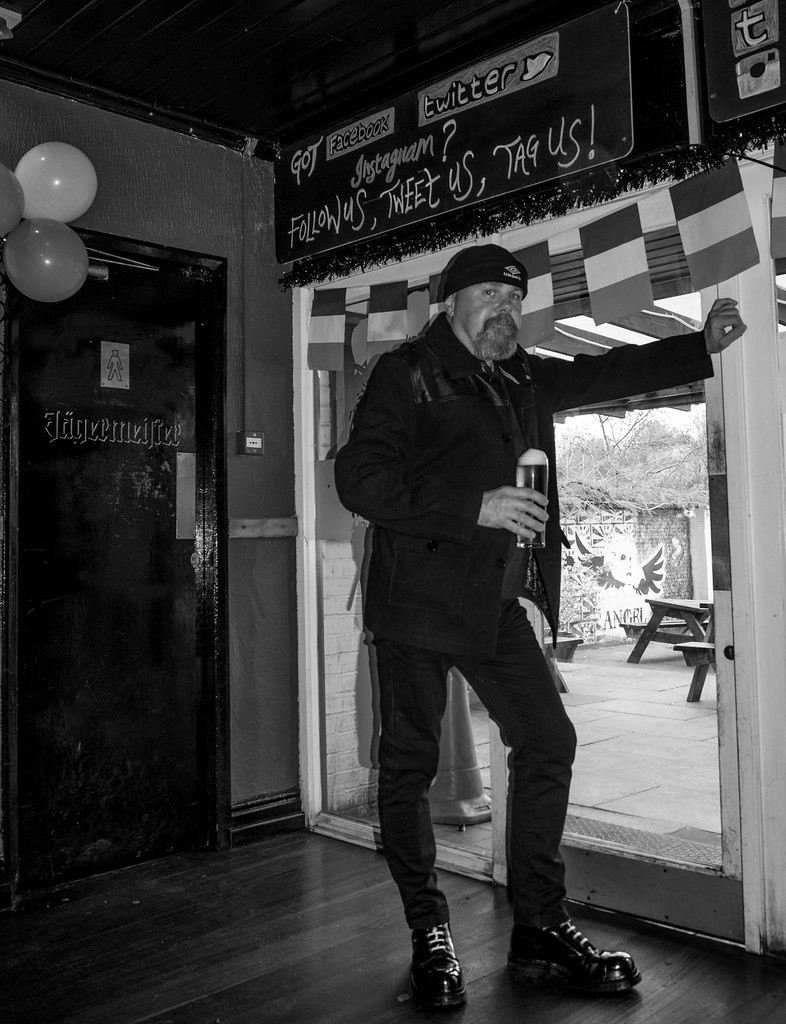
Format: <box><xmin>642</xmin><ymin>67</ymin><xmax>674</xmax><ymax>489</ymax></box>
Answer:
<box><xmin>618</xmin><ymin>619</ymin><xmax>709</xmax><ymax>634</ymax></box>
<box><xmin>542</xmin><ymin>635</ymin><xmax>585</xmax><ymax>664</ymax></box>
<box><xmin>673</xmin><ymin>640</ymin><xmax>717</xmax><ymax>671</ymax></box>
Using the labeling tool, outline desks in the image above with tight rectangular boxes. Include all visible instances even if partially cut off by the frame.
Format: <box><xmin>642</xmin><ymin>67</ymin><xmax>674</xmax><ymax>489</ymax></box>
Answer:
<box><xmin>625</xmin><ymin>598</ymin><xmax>715</xmax><ymax>702</ymax></box>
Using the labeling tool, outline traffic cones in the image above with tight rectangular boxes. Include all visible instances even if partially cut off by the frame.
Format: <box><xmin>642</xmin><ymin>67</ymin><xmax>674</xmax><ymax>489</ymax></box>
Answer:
<box><xmin>428</xmin><ymin>668</ymin><xmax>493</xmax><ymax>825</ymax></box>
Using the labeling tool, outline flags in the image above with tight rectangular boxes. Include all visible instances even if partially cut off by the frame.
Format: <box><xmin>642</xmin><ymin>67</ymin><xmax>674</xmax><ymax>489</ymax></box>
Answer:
<box><xmin>308</xmin><ymin>137</ymin><xmax>786</xmax><ymax>374</ymax></box>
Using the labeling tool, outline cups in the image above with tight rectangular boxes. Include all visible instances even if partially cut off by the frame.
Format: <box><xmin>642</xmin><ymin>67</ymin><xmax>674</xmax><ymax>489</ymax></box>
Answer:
<box><xmin>514</xmin><ymin>448</ymin><xmax>548</xmax><ymax>549</ymax></box>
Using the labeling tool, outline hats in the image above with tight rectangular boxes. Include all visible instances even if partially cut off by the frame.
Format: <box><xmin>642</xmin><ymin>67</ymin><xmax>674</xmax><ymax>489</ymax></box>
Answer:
<box><xmin>443</xmin><ymin>244</ymin><xmax>528</xmax><ymax>300</ymax></box>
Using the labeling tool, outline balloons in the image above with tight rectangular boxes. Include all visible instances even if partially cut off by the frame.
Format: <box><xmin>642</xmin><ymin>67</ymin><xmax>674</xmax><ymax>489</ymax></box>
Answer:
<box><xmin>0</xmin><ymin>141</ymin><xmax>97</xmax><ymax>301</ymax></box>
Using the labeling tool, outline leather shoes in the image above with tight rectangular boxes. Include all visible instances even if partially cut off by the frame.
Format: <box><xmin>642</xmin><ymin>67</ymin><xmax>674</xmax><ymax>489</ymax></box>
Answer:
<box><xmin>409</xmin><ymin>920</ymin><xmax>467</xmax><ymax>1006</ymax></box>
<box><xmin>508</xmin><ymin>912</ymin><xmax>641</xmax><ymax>991</ymax></box>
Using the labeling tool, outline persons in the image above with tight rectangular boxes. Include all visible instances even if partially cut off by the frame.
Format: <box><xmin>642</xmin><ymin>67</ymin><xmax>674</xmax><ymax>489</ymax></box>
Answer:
<box><xmin>334</xmin><ymin>248</ymin><xmax>750</xmax><ymax>1008</ymax></box>
